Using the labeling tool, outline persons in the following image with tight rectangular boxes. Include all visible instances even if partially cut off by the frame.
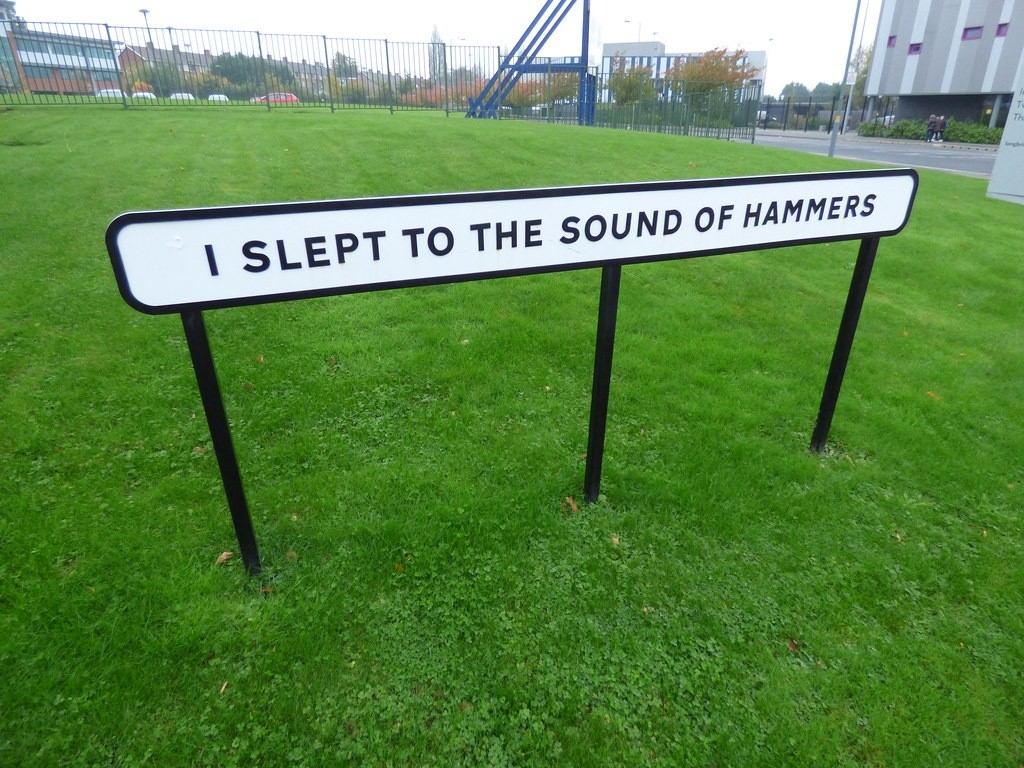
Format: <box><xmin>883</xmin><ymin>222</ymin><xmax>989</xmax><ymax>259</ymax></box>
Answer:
<box><xmin>926</xmin><ymin>115</ymin><xmax>948</xmax><ymax>142</ymax></box>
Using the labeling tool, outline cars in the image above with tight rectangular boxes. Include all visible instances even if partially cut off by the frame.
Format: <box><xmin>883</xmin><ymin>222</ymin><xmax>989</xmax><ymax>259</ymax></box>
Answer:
<box><xmin>132</xmin><ymin>92</ymin><xmax>156</xmax><ymax>98</ymax></box>
<box><xmin>210</xmin><ymin>94</ymin><xmax>229</xmax><ymax>101</ymax></box>
<box><xmin>171</xmin><ymin>93</ymin><xmax>195</xmax><ymax>99</ymax></box>
<box><xmin>250</xmin><ymin>92</ymin><xmax>299</xmax><ymax>103</ymax></box>
<box><xmin>96</xmin><ymin>88</ymin><xmax>128</xmax><ymax>98</ymax></box>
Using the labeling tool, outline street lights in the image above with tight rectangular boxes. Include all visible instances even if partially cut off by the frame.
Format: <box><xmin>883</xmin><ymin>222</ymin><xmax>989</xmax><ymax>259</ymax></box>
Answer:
<box><xmin>138</xmin><ymin>8</ymin><xmax>164</xmax><ymax>97</ymax></box>
<box><xmin>624</xmin><ymin>19</ymin><xmax>642</xmax><ymax>67</ymax></box>
